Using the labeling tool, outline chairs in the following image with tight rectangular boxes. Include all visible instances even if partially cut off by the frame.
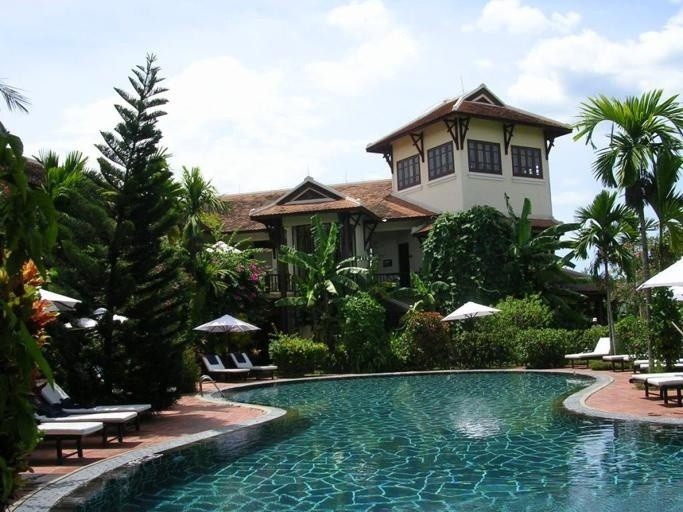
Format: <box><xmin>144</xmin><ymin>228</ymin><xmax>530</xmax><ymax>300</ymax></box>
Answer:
<box><xmin>198</xmin><ymin>350</ymin><xmax>279</xmax><ymax>383</ymax></box>
<box><xmin>565</xmin><ymin>337</ymin><xmax>612</xmax><ymax>369</ymax></box>
<box><xmin>28</xmin><ymin>375</ymin><xmax>153</xmax><ymax>464</ymax></box>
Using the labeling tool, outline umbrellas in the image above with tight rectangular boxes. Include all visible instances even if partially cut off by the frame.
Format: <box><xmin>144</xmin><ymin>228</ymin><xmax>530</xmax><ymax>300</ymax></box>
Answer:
<box><xmin>32</xmin><ymin>287</ymin><xmax>113</xmax><ymax>323</ymax></box>
<box><xmin>58</xmin><ymin>310</ymin><xmax>131</xmax><ymax>332</ymax></box>
<box><xmin>191</xmin><ymin>313</ymin><xmax>262</xmax><ymax>366</ymax></box>
<box><xmin>640</xmin><ymin>284</ymin><xmax>683</xmax><ymax>302</ymax></box>
<box><xmin>440</xmin><ymin>299</ymin><xmax>504</xmax><ymax>363</ymax></box>
<box><xmin>632</xmin><ymin>255</ymin><xmax>683</xmax><ymax>291</ymax></box>
<box><xmin>205</xmin><ymin>240</ymin><xmax>245</xmax><ymax>254</ymax></box>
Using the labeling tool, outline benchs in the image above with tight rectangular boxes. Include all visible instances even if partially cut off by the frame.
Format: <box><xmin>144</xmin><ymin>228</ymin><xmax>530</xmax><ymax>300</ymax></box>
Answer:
<box><xmin>603</xmin><ymin>351</ymin><xmax>682</xmax><ymax>407</ymax></box>
